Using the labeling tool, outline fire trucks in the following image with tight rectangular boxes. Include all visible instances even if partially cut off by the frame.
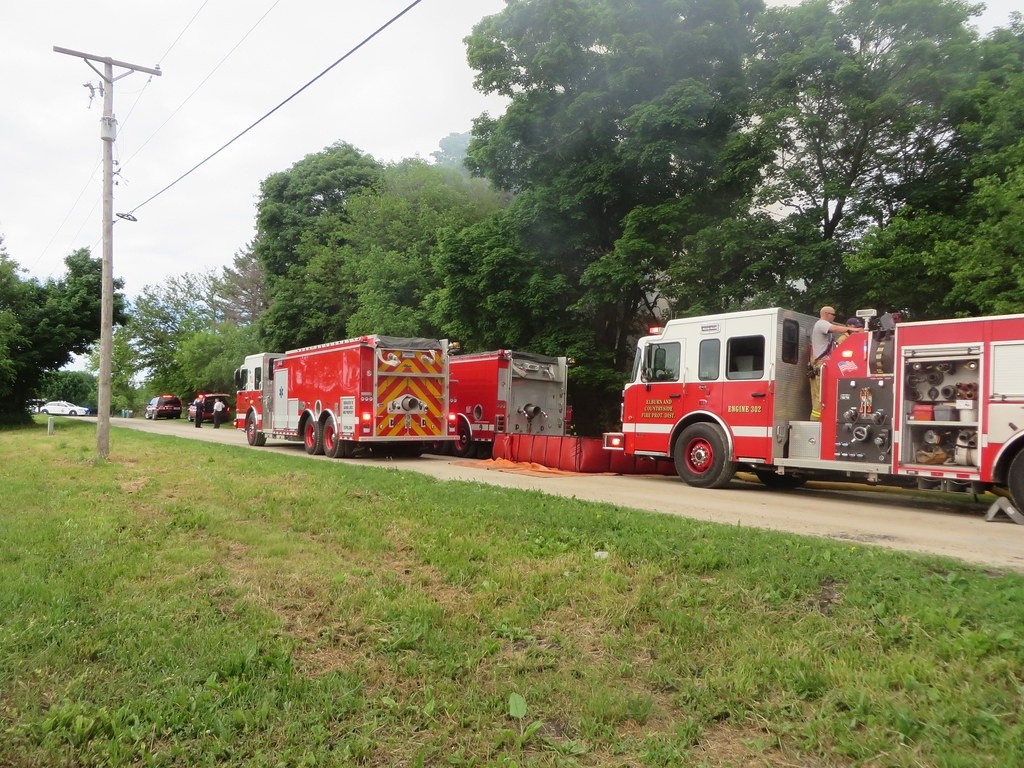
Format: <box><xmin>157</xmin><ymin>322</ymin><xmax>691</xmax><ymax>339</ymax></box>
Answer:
<box><xmin>425</xmin><ymin>349</ymin><xmax>576</xmax><ymax>457</ymax></box>
<box><xmin>602</xmin><ymin>306</ymin><xmax>1024</xmax><ymax>517</ymax></box>
<box><xmin>234</xmin><ymin>334</ymin><xmax>460</xmax><ymax>459</ymax></box>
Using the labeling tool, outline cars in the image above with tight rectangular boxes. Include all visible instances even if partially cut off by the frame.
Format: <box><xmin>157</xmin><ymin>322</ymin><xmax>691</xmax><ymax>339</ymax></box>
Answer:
<box><xmin>36</xmin><ymin>401</ymin><xmax>91</xmax><ymax>416</ymax></box>
<box><xmin>187</xmin><ymin>394</ymin><xmax>231</xmax><ymax>422</ymax></box>
<box><xmin>145</xmin><ymin>396</ymin><xmax>182</xmax><ymax>419</ymax></box>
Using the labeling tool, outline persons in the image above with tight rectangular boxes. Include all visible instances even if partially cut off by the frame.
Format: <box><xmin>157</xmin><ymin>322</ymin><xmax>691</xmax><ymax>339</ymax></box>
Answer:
<box><xmin>212</xmin><ymin>398</ymin><xmax>225</xmax><ymax>428</ymax></box>
<box><xmin>809</xmin><ymin>306</ymin><xmax>863</xmax><ymax>421</ymax></box>
<box><xmin>195</xmin><ymin>396</ymin><xmax>203</xmax><ymax>428</ymax></box>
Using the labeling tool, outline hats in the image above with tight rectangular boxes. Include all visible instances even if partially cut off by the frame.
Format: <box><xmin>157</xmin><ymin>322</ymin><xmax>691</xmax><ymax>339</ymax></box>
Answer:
<box><xmin>846</xmin><ymin>318</ymin><xmax>863</xmax><ymax>327</ymax></box>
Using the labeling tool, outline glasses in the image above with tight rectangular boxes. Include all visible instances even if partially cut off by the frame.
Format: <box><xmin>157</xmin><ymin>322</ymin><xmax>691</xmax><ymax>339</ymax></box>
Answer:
<box><xmin>825</xmin><ymin>312</ymin><xmax>836</xmax><ymax>316</ymax></box>
